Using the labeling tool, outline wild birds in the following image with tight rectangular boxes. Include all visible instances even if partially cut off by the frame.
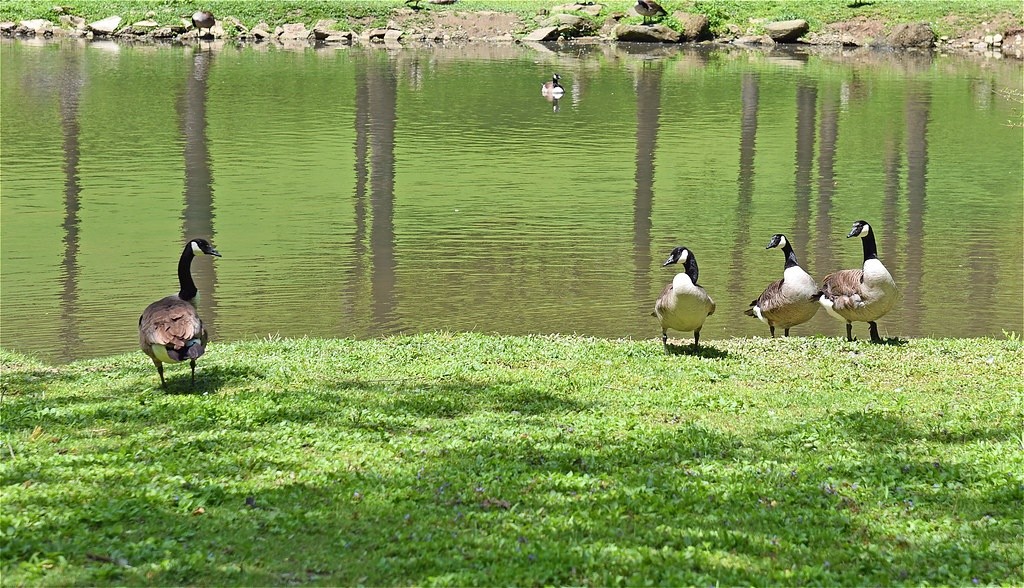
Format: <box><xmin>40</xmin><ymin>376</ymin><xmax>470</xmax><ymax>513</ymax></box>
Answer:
<box><xmin>192</xmin><ymin>11</ymin><xmax>215</xmax><ymax>36</ymax></box>
<box><xmin>634</xmin><ymin>0</ymin><xmax>668</xmax><ymax>27</ymax></box>
<box><xmin>809</xmin><ymin>220</ymin><xmax>898</xmax><ymax>342</ymax></box>
<box><xmin>744</xmin><ymin>233</ymin><xmax>823</xmax><ymax>339</ymax></box>
<box><xmin>542</xmin><ymin>93</ymin><xmax>564</xmax><ymax>112</ymax></box>
<box><xmin>540</xmin><ymin>74</ymin><xmax>565</xmax><ymax>93</ymax></box>
<box><xmin>650</xmin><ymin>246</ymin><xmax>716</xmax><ymax>346</ymax></box>
<box><xmin>138</xmin><ymin>238</ymin><xmax>222</xmax><ymax>387</ymax></box>
